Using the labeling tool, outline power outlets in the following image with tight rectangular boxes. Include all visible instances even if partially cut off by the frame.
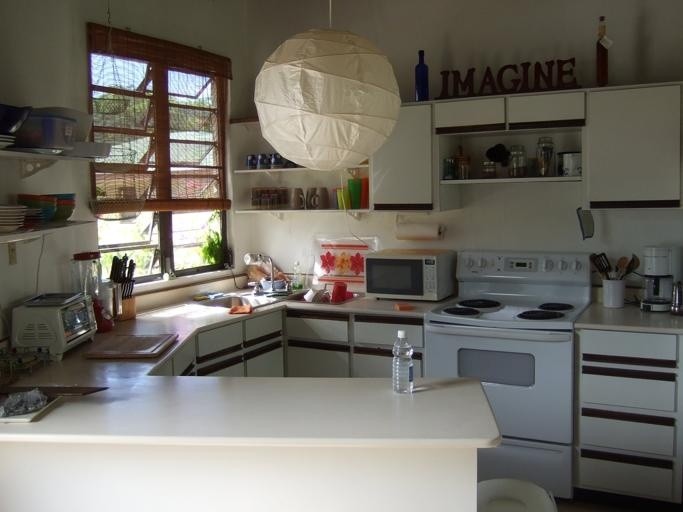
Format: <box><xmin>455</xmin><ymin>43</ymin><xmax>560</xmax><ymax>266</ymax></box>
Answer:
<box><xmin>8</xmin><ymin>242</ymin><xmax>17</xmax><ymax>266</ymax></box>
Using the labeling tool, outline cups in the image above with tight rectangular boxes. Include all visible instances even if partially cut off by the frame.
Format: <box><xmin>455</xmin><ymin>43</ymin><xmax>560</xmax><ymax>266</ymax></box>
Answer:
<box><xmin>332</xmin><ymin>282</ymin><xmax>347</xmax><ymax>303</ymax></box>
<box><xmin>360</xmin><ymin>178</ymin><xmax>369</xmax><ymax>208</ymax></box>
<box><xmin>245</xmin><ymin>152</ymin><xmax>299</xmax><ymax>169</ymax></box>
<box><xmin>347</xmin><ymin>179</ymin><xmax>362</xmax><ymax>209</ymax></box>
<box><xmin>601</xmin><ymin>279</ymin><xmax>625</xmax><ymax>309</ymax></box>
<box><xmin>250</xmin><ymin>186</ymin><xmax>331</xmax><ymax>209</ymax></box>
<box><xmin>98</xmin><ymin>279</ymin><xmax>115</xmax><ymax>319</ymax></box>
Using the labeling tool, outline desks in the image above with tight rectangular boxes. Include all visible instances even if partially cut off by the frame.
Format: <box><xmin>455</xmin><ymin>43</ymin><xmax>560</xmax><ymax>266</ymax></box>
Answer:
<box><xmin>0</xmin><ymin>375</ymin><xmax>501</xmax><ymax>512</ymax></box>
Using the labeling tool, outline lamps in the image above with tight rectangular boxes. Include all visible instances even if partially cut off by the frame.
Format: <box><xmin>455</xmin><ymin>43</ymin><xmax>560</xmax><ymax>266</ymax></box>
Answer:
<box><xmin>253</xmin><ymin>2</ymin><xmax>399</xmax><ymax>174</ymax></box>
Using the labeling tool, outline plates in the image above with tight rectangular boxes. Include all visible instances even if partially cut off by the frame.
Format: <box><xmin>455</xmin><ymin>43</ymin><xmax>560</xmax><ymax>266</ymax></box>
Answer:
<box><xmin>0</xmin><ymin>134</ymin><xmax>15</xmax><ymax>147</ymax></box>
<box><xmin>0</xmin><ymin>203</ymin><xmax>27</xmax><ymax>233</ymax></box>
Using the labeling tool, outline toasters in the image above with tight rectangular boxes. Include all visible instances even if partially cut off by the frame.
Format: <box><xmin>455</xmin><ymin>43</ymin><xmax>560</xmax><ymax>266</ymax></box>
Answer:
<box><xmin>9</xmin><ymin>292</ymin><xmax>98</xmax><ymax>359</ymax></box>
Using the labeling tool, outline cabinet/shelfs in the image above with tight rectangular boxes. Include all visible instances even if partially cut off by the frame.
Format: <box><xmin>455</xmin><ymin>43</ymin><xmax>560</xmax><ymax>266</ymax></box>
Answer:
<box><xmin>284</xmin><ymin>308</ymin><xmax>424</xmax><ymax>377</ymax></box>
<box><xmin>195</xmin><ymin>308</ymin><xmax>284</xmax><ymax>376</ymax></box>
<box><xmin>432</xmin><ymin>86</ymin><xmax>586</xmax><ymax>184</ymax></box>
<box><xmin>150</xmin><ymin>332</ymin><xmax>196</xmax><ymax>376</ymax></box>
<box><xmin>585</xmin><ymin>80</ymin><xmax>683</xmax><ymax>212</ymax></box>
<box><xmin>575</xmin><ymin>301</ymin><xmax>683</xmax><ymax>511</ymax></box>
<box><xmin>228</xmin><ymin>116</ymin><xmax>374</xmax><ymax>214</ymax></box>
<box><xmin>0</xmin><ymin>150</ymin><xmax>99</xmax><ymax>245</ymax></box>
<box><xmin>371</xmin><ymin>100</ymin><xmax>464</xmax><ymax>214</ymax></box>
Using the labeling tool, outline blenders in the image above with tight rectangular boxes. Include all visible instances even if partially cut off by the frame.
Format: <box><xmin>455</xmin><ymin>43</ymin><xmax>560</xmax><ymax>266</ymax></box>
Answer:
<box><xmin>73</xmin><ymin>251</ymin><xmax>115</xmax><ymax>331</ymax></box>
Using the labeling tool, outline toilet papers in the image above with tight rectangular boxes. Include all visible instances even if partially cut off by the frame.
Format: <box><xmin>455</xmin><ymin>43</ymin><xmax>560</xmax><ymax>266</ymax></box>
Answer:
<box><xmin>394</xmin><ymin>221</ymin><xmax>444</xmax><ymax>242</ymax></box>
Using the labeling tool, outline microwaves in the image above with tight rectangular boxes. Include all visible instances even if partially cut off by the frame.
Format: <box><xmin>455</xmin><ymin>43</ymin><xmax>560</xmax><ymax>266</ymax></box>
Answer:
<box><xmin>421</xmin><ymin>320</ymin><xmax>578</xmax><ymax>501</ymax></box>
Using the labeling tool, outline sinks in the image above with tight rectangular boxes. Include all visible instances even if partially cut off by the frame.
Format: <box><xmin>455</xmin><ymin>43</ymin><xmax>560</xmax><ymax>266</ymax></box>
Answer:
<box><xmin>282</xmin><ymin>289</ymin><xmax>365</xmax><ymax>304</ymax></box>
<box><xmin>193</xmin><ymin>291</ymin><xmax>282</xmax><ymax>312</ymax></box>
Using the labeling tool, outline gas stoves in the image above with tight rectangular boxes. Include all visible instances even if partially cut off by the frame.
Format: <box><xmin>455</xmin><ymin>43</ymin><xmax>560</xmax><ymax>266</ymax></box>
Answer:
<box><xmin>442</xmin><ymin>295</ymin><xmax>577</xmax><ymax>321</ymax></box>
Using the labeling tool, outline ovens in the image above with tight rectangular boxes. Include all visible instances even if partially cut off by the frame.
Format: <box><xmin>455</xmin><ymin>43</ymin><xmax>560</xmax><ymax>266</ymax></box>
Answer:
<box><xmin>364</xmin><ymin>249</ymin><xmax>458</xmax><ymax>300</ymax></box>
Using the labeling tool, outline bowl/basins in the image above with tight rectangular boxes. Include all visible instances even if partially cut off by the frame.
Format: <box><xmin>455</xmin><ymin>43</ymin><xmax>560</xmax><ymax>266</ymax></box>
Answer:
<box><xmin>18</xmin><ymin>190</ymin><xmax>78</xmax><ymax>225</ymax></box>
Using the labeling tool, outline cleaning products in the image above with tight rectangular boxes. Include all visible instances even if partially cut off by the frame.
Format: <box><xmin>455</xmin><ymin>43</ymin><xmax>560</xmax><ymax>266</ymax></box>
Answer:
<box><xmin>290</xmin><ymin>260</ymin><xmax>304</xmax><ymax>289</ymax></box>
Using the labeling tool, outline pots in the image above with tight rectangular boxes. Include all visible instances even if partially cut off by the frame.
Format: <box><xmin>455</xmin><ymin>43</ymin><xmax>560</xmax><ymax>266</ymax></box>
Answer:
<box><xmin>0</xmin><ymin>104</ymin><xmax>32</xmax><ymax>136</ymax></box>
<box><xmin>557</xmin><ymin>151</ymin><xmax>581</xmax><ymax>177</ymax></box>
<box><xmin>20</xmin><ymin>115</ymin><xmax>77</xmax><ymax>149</ymax></box>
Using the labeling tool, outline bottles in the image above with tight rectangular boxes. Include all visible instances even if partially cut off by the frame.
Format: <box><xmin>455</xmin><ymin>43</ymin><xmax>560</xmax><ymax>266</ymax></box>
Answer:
<box><xmin>414</xmin><ymin>49</ymin><xmax>429</xmax><ymax>101</ymax></box>
<box><xmin>443</xmin><ymin>155</ymin><xmax>467</xmax><ymax>180</ymax></box>
<box><xmin>509</xmin><ymin>144</ymin><xmax>527</xmax><ymax>178</ymax></box>
<box><xmin>536</xmin><ymin>136</ymin><xmax>556</xmax><ymax>176</ymax></box>
<box><xmin>392</xmin><ymin>330</ymin><xmax>415</xmax><ymax>395</ymax></box>
<box><xmin>292</xmin><ymin>261</ymin><xmax>304</xmax><ymax>289</ymax></box>
<box><xmin>672</xmin><ymin>282</ymin><xmax>683</xmax><ymax>315</ymax></box>
<box><xmin>595</xmin><ymin>16</ymin><xmax>609</xmax><ymax>88</ymax></box>
<box><xmin>478</xmin><ymin>162</ymin><xmax>497</xmax><ymax>178</ymax></box>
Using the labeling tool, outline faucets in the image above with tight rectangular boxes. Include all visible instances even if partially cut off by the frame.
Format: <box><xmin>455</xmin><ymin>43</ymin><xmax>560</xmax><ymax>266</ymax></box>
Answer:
<box><xmin>257</xmin><ymin>253</ymin><xmax>274</xmax><ymax>288</ymax></box>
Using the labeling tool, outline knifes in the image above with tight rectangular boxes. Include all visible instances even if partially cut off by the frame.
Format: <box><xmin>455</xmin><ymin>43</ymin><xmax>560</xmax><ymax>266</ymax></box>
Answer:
<box><xmin>110</xmin><ymin>254</ymin><xmax>135</xmax><ymax>299</ymax></box>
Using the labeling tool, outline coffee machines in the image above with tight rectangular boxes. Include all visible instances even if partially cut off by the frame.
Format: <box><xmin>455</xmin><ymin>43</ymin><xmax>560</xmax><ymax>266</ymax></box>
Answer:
<box><xmin>638</xmin><ymin>244</ymin><xmax>682</xmax><ymax>311</ymax></box>
